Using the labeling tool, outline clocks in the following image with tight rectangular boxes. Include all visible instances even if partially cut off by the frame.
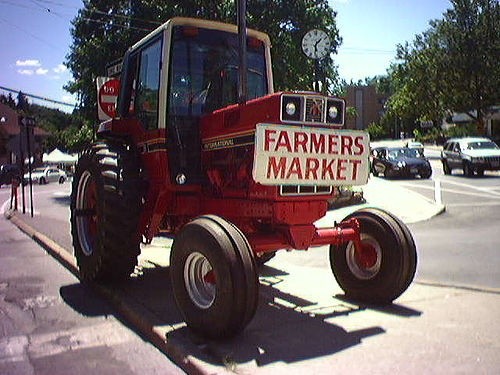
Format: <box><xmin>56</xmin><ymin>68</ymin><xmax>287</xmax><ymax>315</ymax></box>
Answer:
<box><xmin>298</xmin><ymin>28</ymin><xmax>331</xmax><ymax>59</ymax></box>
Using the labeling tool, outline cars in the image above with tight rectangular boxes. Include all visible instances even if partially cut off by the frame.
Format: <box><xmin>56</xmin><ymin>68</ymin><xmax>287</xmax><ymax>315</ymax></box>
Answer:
<box><xmin>0</xmin><ymin>163</ymin><xmax>26</xmax><ymax>187</ymax></box>
<box><xmin>371</xmin><ymin>147</ymin><xmax>432</xmax><ymax>179</ymax></box>
<box><xmin>405</xmin><ymin>142</ymin><xmax>423</xmax><ymax>152</ymax></box>
<box><xmin>24</xmin><ymin>167</ymin><xmax>67</xmax><ymax>183</ymax></box>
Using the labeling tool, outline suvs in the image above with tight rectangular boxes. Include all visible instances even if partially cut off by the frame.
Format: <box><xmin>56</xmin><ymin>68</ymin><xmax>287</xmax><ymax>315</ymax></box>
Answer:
<box><xmin>441</xmin><ymin>137</ymin><xmax>500</xmax><ymax>176</ymax></box>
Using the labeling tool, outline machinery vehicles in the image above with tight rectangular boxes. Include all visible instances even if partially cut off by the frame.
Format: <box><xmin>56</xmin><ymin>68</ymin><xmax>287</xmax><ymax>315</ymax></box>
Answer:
<box><xmin>70</xmin><ymin>0</ymin><xmax>417</xmax><ymax>338</ymax></box>
